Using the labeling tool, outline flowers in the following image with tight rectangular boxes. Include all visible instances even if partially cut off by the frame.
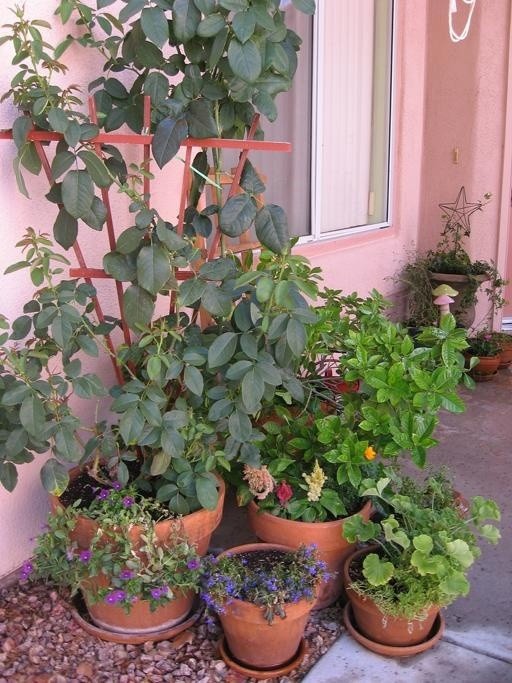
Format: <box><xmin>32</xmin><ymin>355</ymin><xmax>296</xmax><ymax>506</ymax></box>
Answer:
<box><xmin>231</xmin><ymin>436</ymin><xmax>390</xmax><ymax>530</ymax></box>
<box><xmin>464</xmin><ymin>331</ymin><xmax>506</xmax><ymax>358</ymax></box>
<box><xmin>196</xmin><ymin>541</ymin><xmax>338</xmax><ymax>622</ymax></box>
<box><xmin>20</xmin><ymin>484</ymin><xmax>216</xmax><ymax>616</ymax></box>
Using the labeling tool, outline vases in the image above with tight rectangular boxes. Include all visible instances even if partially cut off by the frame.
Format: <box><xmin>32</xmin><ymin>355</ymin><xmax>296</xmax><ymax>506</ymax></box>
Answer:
<box><xmin>461</xmin><ymin>347</ymin><xmax>500</xmax><ymax>380</ymax></box>
<box><xmin>199</xmin><ymin>543</ymin><xmax>321</xmax><ymax>667</ymax></box>
<box><xmin>245</xmin><ymin>497</ymin><xmax>379</xmax><ymax>612</ymax></box>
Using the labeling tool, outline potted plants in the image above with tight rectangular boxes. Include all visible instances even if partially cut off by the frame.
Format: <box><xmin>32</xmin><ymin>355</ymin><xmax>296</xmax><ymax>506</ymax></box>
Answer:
<box><xmin>409</xmin><ymin>211</ymin><xmax>506</xmax><ymax>328</ymax></box>
<box><xmin>384</xmin><ymin>470</ymin><xmax>470</xmax><ymax>530</ymax></box>
<box><xmin>238</xmin><ymin>237</ymin><xmax>471</xmax><ymax>467</ymax></box>
<box><xmin>1</xmin><ymin>0</ymin><xmax>321</xmax><ymax>639</ymax></box>
<box><xmin>486</xmin><ymin>332</ymin><xmax>512</xmax><ymax>365</ymax></box>
<box><xmin>335</xmin><ymin>476</ymin><xmax>505</xmax><ymax>658</ymax></box>
